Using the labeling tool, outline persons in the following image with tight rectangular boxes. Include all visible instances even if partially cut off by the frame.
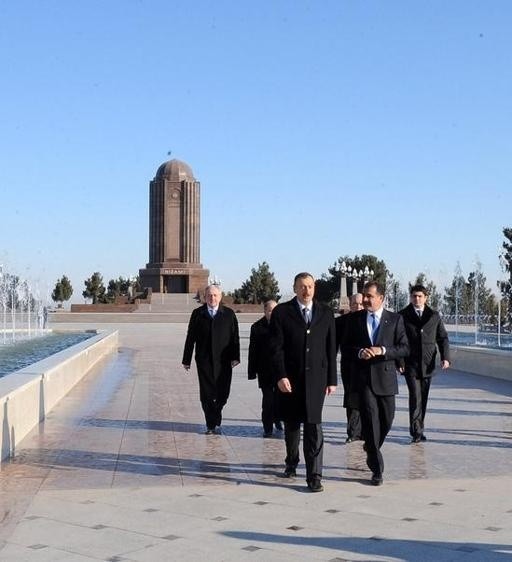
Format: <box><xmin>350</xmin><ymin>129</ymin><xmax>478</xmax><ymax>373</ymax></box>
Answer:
<box><xmin>182</xmin><ymin>285</ymin><xmax>240</xmax><ymax>433</ymax></box>
<box><xmin>394</xmin><ymin>285</ymin><xmax>451</xmax><ymax>444</ymax></box>
<box><xmin>333</xmin><ymin>292</ymin><xmax>365</xmax><ymax>443</ymax></box>
<box><xmin>269</xmin><ymin>271</ymin><xmax>338</xmax><ymax>493</ymax></box>
<box><xmin>248</xmin><ymin>300</ymin><xmax>283</xmax><ymax>437</ymax></box>
<box><xmin>340</xmin><ymin>282</ymin><xmax>410</xmax><ymax>486</ymax></box>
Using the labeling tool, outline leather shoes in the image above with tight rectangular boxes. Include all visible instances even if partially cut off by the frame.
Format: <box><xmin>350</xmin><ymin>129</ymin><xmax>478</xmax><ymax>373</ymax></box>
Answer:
<box><xmin>216</xmin><ymin>418</ymin><xmax>221</xmax><ymax>427</ymax></box>
<box><xmin>274</xmin><ymin>420</ymin><xmax>283</xmax><ymax>432</ymax></box>
<box><xmin>346</xmin><ymin>436</ymin><xmax>352</xmax><ymax>444</ymax></box>
<box><xmin>276</xmin><ymin>471</ymin><xmax>296</xmax><ymax>478</ymax></box>
<box><xmin>204</xmin><ymin>429</ymin><xmax>215</xmax><ymax>434</ymax></box>
<box><xmin>421</xmin><ymin>434</ymin><xmax>426</xmax><ymax>440</ymax></box>
<box><xmin>371</xmin><ymin>471</ymin><xmax>383</xmax><ymax>486</ymax></box>
<box><xmin>308</xmin><ymin>477</ymin><xmax>321</xmax><ymax>492</ymax></box>
<box><xmin>263</xmin><ymin>432</ymin><xmax>273</xmax><ymax>438</ymax></box>
<box><xmin>409</xmin><ymin>437</ymin><xmax>421</xmax><ymax>445</ymax></box>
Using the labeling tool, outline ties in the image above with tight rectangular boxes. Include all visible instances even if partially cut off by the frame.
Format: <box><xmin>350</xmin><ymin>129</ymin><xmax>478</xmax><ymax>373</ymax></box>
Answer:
<box><xmin>416</xmin><ymin>310</ymin><xmax>423</xmax><ymax>318</ymax></box>
<box><xmin>371</xmin><ymin>312</ymin><xmax>379</xmax><ymax>349</ymax></box>
<box><xmin>302</xmin><ymin>308</ymin><xmax>310</xmax><ymax>328</ymax></box>
<box><xmin>209</xmin><ymin>309</ymin><xmax>214</xmax><ymax>319</ymax></box>
